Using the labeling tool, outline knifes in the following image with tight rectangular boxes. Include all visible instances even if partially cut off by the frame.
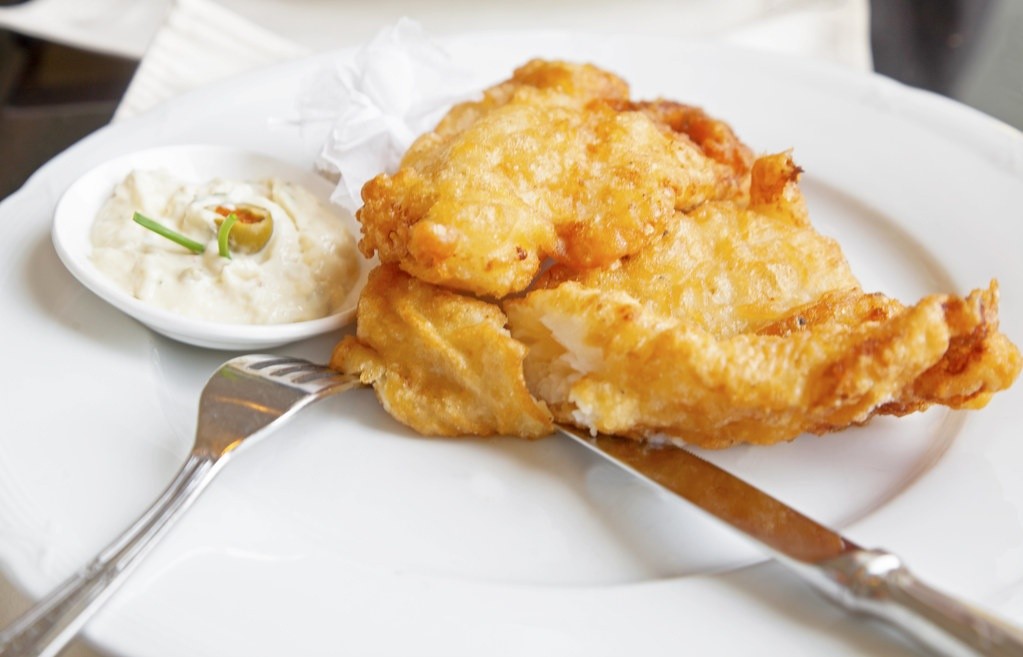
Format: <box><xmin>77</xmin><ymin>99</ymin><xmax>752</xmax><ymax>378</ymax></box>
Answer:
<box><xmin>554</xmin><ymin>425</ymin><xmax>1023</xmax><ymax>656</ymax></box>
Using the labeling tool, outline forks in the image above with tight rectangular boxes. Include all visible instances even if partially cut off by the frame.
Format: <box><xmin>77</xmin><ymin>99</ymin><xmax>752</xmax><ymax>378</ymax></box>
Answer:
<box><xmin>1</xmin><ymin>353</ymin><xmax>374</xmax><ymax>656</ymax></box>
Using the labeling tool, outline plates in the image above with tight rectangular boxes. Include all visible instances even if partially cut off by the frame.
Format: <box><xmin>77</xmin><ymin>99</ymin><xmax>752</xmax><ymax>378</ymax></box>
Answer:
<box><xmin>2</xmin><ymin>76</ymin><xmax>1023</xmax><ymax>656</ymax></box>
<box><xmin>52</xmin><ymin>146</ymin><xmax>381</xmax><ymax>352</ymax></box>
